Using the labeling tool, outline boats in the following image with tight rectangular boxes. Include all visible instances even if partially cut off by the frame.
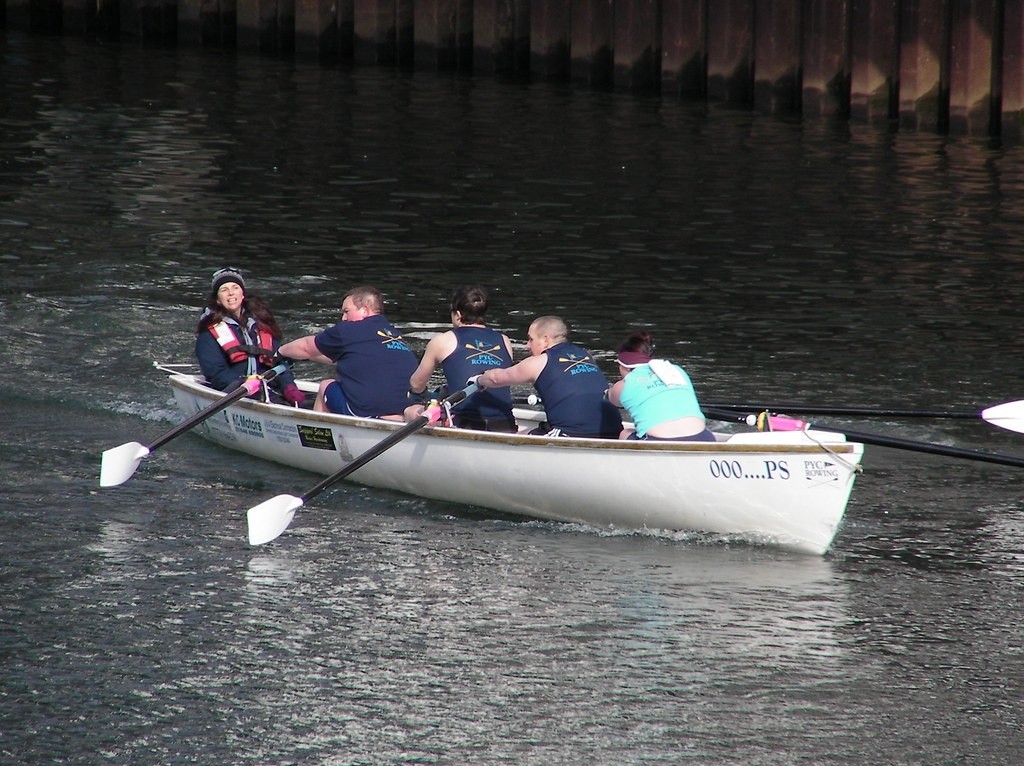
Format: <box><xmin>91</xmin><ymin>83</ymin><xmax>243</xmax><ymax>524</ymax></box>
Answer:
<box><xmin>153</xmin><ymin>361</ymin><xmax>866</xmax><ymax>555</ymax></box>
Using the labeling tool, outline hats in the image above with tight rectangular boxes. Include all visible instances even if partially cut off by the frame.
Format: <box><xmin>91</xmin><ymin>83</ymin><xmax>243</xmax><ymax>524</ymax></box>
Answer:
<box><xmin>210</xmin><ymin>266</ymin><xmax>246</xmax><ymax>292</ymax></box>
<box><xmin>613</xmin><ymin>351</ymin><xmax>651</xmax><ymax>369</ymax></box>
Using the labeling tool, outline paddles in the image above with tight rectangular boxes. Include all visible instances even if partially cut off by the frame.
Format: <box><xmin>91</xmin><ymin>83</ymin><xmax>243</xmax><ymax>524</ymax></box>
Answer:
<box><xmin>247</xmin><ymin>369</ymin><xmax>492</xmax><ymax>545</ymax></box>
<box><xmin>99</xmin><ymin>358</ymin><xmax>295</xmax><ymax>487</ymax></box>
<box><xmin>521</xmin><ymin>388</ymin><xmax>1024</xmax><ymax>465</ymax></box>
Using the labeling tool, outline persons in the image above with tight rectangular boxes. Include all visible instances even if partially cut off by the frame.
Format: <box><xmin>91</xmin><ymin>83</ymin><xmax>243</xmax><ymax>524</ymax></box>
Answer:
<box><xmin>605</xmin><ymin>333</ymin><xmax>717</xmax><ymax>441</ymax></box>
<box><xmin>403</xmin><ymin>286</ymin><xmax>519</xmax><ymax>433</ymax></box>
<box><xmin>467</xmin><ymin>317</ymin><xmax>623</xmax><ymax>440</ymax></box>
<box><xmin>196</xmin><ymin>269</ymin><xmax>314</xmax><ymax>407</ymax></box>
<box><xmin>272</xmin><ymin>287</ymin><xmax>419</xmax><ymax>418</ymax></box>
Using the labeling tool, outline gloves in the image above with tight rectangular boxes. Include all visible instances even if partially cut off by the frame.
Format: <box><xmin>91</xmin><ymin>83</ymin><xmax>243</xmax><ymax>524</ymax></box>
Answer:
<box><xmin>271</xmin><ymin>343</ymin><xmax>294</xmax><ymax>368</ymax></box>
<box><xmin>406</xmin><ymin>386</ymin><xmax>428</xmax><ymax>398</ymax></box>
<box><xmin>282</xmin><ymin>384</ymin><xmax>305</xmax><ymax>404</ymax></box>
<box><xmin>465</xmin><ymin>373</ymin><xmax>487</xmax><ymax>393</ymax></box>
